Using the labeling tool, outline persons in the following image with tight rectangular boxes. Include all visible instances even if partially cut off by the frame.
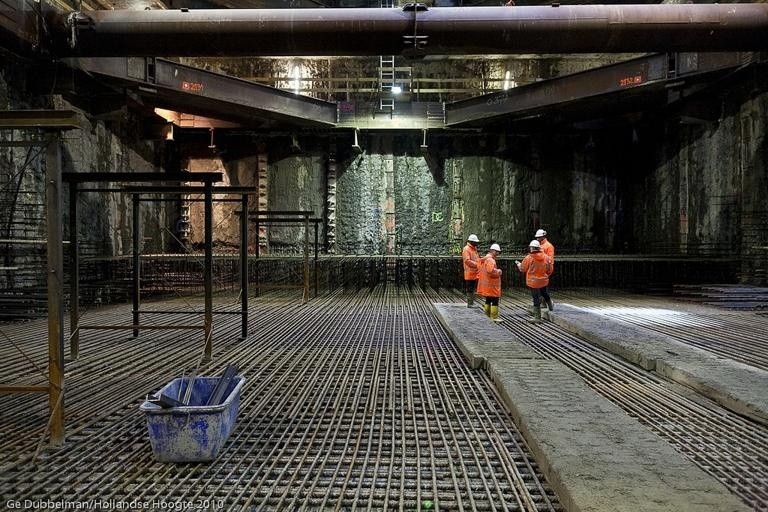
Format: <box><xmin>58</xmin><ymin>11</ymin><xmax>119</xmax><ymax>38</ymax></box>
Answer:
<box><xmin>515</xmin><ymin>228</ymin><xmax>555</xmax><ymax>324</ymax></box>
<box><xmin>462</xmin><ymin>233</ymin><xmax>481</xmax><ymax>308</ymax></box>
<box><xmin>476</xmin><ymin>243</ymin><xmax>505</xmax><ymax>322</ymax></box>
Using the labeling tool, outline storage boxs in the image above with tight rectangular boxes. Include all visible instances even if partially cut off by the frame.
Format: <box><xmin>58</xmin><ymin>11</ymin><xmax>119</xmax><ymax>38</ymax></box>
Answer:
<box><xmin>137</xmin><ymin>376</ymin><xmax>244</xmax><ymax>464</ymax></box>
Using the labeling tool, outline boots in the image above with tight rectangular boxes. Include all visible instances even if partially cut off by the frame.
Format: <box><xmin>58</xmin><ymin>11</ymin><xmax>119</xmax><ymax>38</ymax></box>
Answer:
<box><xmin>529</xmin><ymin>307</ymin><xmax>541</xmax><ymax>323</ymax></box>
<box><xmin>539</xmin><ymin>296</ymin><xmax>554</xmax><ymax>311</ymax></box>
<box><xmin>467</xmin><ymin>293</ymin><xmax>479</xmax><ymax>308</ymax></box>
<box><xmin>484</xmin><ymin>304</ymin><xmax>503</xmax><ymax>322</ymax></box>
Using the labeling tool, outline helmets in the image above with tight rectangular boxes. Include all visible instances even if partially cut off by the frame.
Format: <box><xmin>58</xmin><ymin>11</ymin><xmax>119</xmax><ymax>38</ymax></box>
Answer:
<box><xmin>535</xmin><ymin>229</ymin><xmax>547</xmax><ymax>237</ymax></box>
<box><xmin>468</xmin><ymin>234</ymin><xmax>479</xmax><ymax>242</ymax></box>
<box><xmin>529</xmin><ymin>240</ymin><xmax>540</xmax><ymax>247</ymax></box>
<box><xmin>490</xmin><ymin>244</ymin><xmax>501</xmax><ymax>252</ymax></box>
